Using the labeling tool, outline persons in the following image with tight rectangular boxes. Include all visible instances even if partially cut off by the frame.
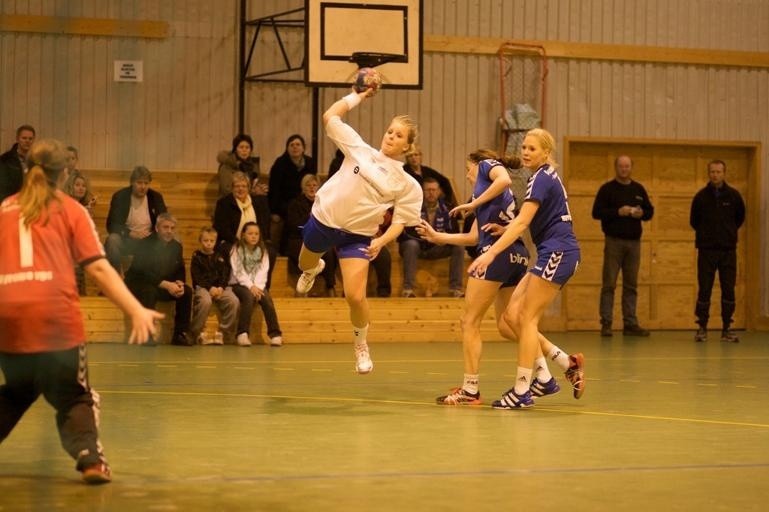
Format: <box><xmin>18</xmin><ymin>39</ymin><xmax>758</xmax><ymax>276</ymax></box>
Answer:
<box><xmin>1</xmin><ymin>127</ymin><xmax>36</xmax><ymax>201</ymax></box>
<box><xmin>467</xmin><ymin>127</ymin><xmax>581</xmax><ymax>410</ymax></box>
<box><xmin>124</xmin><ymin>211</ymin><xmax>284</xmax><ymax>346</ymax></box>
<box><xmin>592</xmin><ymin>154</ymin><xmax>654</xmax><ymax>337</ymax></box>
<box><xmin>0</xmin><ymin>138</ymin><xmax>166</xmax><ymax>482</ymax></box>
<box><xmin>296</xmin><ymin>85</ymin><xmax>423</xmax><ymax>372</ymax></box>
<box><xmin>209</xmin><ymin>125</ymin><xmax>337</xmax><ymax>297</ymax></box>
<box><xmin>415</xmin><ymin>148</ymin><xmax>585</xmax><ymax>406</ymax></box>
<box><xmin>64</xmin><ymin>146</ymin><xmax>98</xmax><ymax>209</ymax></box>
<box><xmin>105</xmin><ymin>166</ymin><xmax>168</xmax><ymax>278</ymax></box>
<box><xmin>370</xmin><ymin>177</ymin><xmax>465</xmax><ymax>300</ymax></box>
<box><xmin>690</xmin><ymin>161</ymin><xmax>745</xmax><ymax>343</ymax></box>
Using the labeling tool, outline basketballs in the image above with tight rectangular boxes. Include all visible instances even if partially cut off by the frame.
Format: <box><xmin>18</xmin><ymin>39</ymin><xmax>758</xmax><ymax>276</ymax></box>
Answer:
<box><xmin>352</xmin><ymin>66</ymin><xmax>382</xmax><ymax>96</ymax></box>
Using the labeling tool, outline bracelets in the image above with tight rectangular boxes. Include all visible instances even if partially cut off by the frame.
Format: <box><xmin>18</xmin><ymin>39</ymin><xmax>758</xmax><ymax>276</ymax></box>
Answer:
<box><xmin>343</xmin><ymin>92</ymin><xmax>361</xmax><ymax>110</ymax></box>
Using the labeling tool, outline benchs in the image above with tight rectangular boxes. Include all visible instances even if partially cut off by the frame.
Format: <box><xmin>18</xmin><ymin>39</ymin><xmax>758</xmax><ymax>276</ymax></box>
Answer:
<box><xmin>65</xmin><ymin>166</ymin><xmax>517</xmax><ymax>347</ymax></box>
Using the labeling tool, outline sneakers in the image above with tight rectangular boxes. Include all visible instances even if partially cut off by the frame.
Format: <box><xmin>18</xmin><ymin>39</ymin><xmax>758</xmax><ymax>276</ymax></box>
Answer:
<box><xmin>272</xmin><ymin>337</ymin><xmax>282</xmax><ymax>344</ymax></box>
<box><xmin>695</xmin><ymin>327</ymin><xmax>707</xmax><ymax>341</ymax></box>
<box><xmin>436</xmin><ymin>388</ymin><xmax>483</xmax><ymax>405</ymax></box>
<box><xmin>235</xmin><ymin>333</ymin><xmax>251</xmax><ymax>346</ymax></box>
<box><xmin>296</xmin><ymin>259</ymin><xmax>325</xmax><ymax>295</ymax></box>
<box><xmin>449</xmin><ymin>290</ymin><xmax>465</xmax><ymax>297</ymax></box>
<box><xmin>721</xmin><ymin>330</ymin><xmax>739</xmax><ymax>342</ymax></box>
<box><xmin>83</xmin><ymin>461</ymin><xmax>110</xmax><ymax>482</ymax></box>
<box><xmin>564</xmin><ymin>352</ymin><xmax>585</xmax><ymax>399</ymax></box>
<box><xmin>172</xmin><ymin>330</ymin><xmax>225</xmax><ymax>344</ymax></box>
<box><xmin>530</xmin><ymin>376</ymin><xmax>561</xmax><ymax>398</ymax></box>
<box><xmin>493</xmin><ymin>386</ymin><xmax>536</xmax><ymax>409</ymax></box>
<box><xmin>402</xmin><ymin>289</ymin><xmax>416</xmax><ymax>297</ymax></box>
<box><xmin>354</xmin><ymin>343</ymin><xmax>373</xmax><ymax>374</ymax></box>
<box><xmin>624</xmin><ymin>324</ymin><xmax>649</xmax><ymax>336</ymax></box>
<box><xmin>601</xmin><ymin>321</ymin><xmax>612</xmax><ymax>336</ymax></box>
